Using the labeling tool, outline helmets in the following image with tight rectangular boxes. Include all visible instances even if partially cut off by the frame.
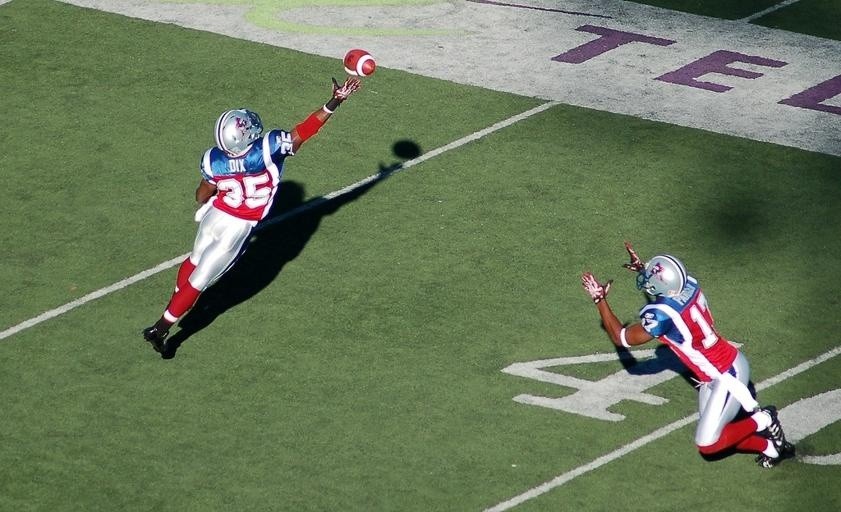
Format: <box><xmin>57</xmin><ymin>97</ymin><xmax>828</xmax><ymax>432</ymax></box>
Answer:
<box><xmin>636</xmin><ymin>254</ymin><xmax>687</xmax><ymax>299</ymax></box>
<box><xmin>213</xmin><ymin>108</ymin><xmax>264</xmax><ymax>160</ymax></box>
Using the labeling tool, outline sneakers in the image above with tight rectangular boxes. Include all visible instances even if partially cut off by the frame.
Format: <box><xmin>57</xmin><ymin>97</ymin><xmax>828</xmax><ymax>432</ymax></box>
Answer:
<box><xmin>756</xmin><ymin>405</ymin><xmax>785</xmax><ymax>449</ymax></box>
<box><xmin>755</xmin><ymin>441</ymin><xmax>796</xmax><ymax>469</ymax></box>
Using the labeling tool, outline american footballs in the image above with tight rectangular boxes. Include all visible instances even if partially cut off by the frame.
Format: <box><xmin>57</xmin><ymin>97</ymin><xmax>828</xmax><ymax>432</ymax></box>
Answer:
<box><xmin>343</xmin><ymin>50</ymin><xmax>376</xmax><ymax>77</ymax></box>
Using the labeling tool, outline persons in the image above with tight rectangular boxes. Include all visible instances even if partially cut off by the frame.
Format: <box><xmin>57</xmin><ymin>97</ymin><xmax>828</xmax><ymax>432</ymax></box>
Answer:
<box><xmin>143</xmin><ymin>73</ymin><xmax>362</xmax><ymax>353</ymax></box>
<box><xmin>581</xmin><ymin>240</ymin><xmax>795</xmax><ymax>469</ymax></box>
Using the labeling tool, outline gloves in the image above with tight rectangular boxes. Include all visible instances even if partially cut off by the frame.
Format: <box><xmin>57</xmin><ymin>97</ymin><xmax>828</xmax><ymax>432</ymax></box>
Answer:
<box><xmin>330</xmin><ymin>76</ymin><xmax>362</xmax><ymax>105</ymax></box>
<box><xmin>580</xmin><ymin>271</ymin><xmax>613</xmax><ymax>305</ymax></box>
<box><xmin>621</xmin><ymin>241</ymin><xmax>644</xmax><ymax>272</ymax></box>
<box><xmin>142</xmin><ymin>326</ymin><xmax>170</xmax><ymax>354</ymax></box>
